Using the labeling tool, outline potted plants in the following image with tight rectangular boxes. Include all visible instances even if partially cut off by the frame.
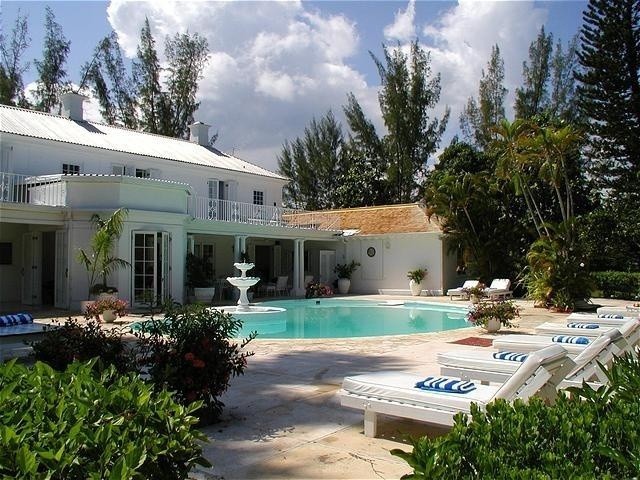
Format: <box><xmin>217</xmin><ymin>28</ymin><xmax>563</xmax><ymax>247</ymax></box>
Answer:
<box><xmin>186</xmin><ymin>252</ymin><xmax>216</xmax><ymax>306</ymax></box>
<box><xmin>407</xmin><ymin>267</ymin><xmax>427</xmax><ymax>297</ymax></box>
<box><xmin>332</xmin><ymin>259</ymin><xmax>362</xmax><ymax>294</ymax></box>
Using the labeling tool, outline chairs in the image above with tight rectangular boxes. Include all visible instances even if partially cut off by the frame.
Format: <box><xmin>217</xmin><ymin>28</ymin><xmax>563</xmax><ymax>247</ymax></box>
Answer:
<box><xmin>435</xmin><ymin>305</ymin><xmax>639</xmax><ymax>399</ymax></box>
<box><xmin>447</xmin><ymin>279</ymin><xmax>513</xmax><ymax>303</ymax></box>
<box><xmin>340</xmin><ymin>343</ymin><xmax>567</xmax><ymax>439</ymax></box>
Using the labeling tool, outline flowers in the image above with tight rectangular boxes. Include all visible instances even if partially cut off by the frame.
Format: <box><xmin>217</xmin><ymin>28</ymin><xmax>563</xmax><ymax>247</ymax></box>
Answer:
<box><xmin>463</xmin><ymin>284</ymin><xmax>486</xmax><ymax>296</ymax></box>
<box><xmin>83</xmin><ymin>299</ymin><xmax>129</xmax><ymax>323</ymax></box>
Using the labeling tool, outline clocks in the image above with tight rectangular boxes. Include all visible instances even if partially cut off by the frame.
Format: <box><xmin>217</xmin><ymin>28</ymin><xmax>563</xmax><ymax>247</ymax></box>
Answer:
<box><xmin>367</xmin><ymin>247</ymin><xmax>376</xmax><ymax>258</ymax></box>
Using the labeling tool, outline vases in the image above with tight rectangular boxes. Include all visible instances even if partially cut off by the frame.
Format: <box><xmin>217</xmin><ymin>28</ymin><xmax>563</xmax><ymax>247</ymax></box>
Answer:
<box><xmin>469</xmin><ymin>294</ymin><xmax>479</xmax><ymax>301</ymax></box>
<box><xmin>103</xmin><ymin>311</ymin><xmax>118</xmax><ymax>322</ymax></box>
